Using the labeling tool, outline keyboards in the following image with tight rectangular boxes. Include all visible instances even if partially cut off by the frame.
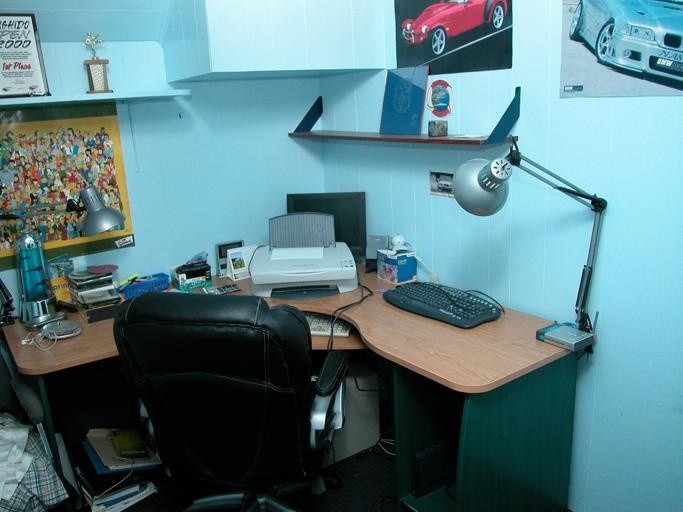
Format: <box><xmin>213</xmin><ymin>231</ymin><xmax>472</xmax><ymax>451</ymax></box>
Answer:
<box><xmin>304</xmin><ymin>314</ymin><xmax>350</xmax><ymax>338</ymax></box>
<box><xmin>383</xmin><ymin>281</ymin><xmax>502</xmax><ymax>329</ymax></box>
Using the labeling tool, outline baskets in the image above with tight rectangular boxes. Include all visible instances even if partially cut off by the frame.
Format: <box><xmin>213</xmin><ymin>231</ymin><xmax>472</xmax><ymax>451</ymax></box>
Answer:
<box><xmin>124</xmin><ymin>273</ymin><xmax>169</xmax><ymax>299</ymax></box>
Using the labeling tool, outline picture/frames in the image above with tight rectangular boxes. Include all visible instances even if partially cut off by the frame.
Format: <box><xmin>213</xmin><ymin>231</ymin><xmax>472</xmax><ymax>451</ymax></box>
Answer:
<box><xmin>0</xmin><ymin>13</ymin><xmax>50</xmax><ymax>98</ymax></box>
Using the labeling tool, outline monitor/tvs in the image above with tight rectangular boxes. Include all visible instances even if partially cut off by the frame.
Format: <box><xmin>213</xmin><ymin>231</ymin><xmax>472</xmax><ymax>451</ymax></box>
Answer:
<box><xmin>287</xmin><ymin>192</ymin><xmax>366</xmax><ymax>263</ymax></box>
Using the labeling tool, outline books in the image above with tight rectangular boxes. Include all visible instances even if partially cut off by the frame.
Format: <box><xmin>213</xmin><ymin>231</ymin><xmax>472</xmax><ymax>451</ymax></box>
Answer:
<box><xmin>80</xmin><ymin>288</ymin><xmax>120</xmax><ymax>310</ymax></box>
<box><xmin>70</xmin><ymin>426</ymin><xmax>161</xmax><ymax>512</ymax></box>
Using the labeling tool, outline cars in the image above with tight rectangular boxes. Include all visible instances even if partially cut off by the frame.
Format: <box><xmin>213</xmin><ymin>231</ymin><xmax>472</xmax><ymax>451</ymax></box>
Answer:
<box><xmin>569</xmin><ymin>0</ymin><xmax>682</xmax><ymax>89</ymax></box>
<box><xmin>400</xmin><ymin>0</ymin><xmax>507</xmax><ymax>55</ymax></box>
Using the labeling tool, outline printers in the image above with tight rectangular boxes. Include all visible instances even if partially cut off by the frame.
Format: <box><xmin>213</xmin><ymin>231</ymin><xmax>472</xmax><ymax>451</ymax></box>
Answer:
<box><xmin>248</xmin><ymin>211</ymin><xmax>359</xmax><ymax>298</ymax></box>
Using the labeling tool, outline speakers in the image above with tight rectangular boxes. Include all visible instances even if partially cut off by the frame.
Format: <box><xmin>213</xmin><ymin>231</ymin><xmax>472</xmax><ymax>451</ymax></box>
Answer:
<box><xmin>365</xmin><ymin>235</ymin><xmax>389</xmax><ymax>273</ymax></box>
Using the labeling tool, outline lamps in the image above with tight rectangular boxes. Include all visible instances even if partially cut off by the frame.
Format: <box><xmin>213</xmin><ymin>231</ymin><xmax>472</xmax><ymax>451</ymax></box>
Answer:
<box><xmin>453</xmin><ymin>147</ymin><xmax>606</xmax><ymax>355</ymax></box>
<box><xmin>0</xmin><ymin>187</ymin><xmax>125</xmax><ymax>237</ymax></box>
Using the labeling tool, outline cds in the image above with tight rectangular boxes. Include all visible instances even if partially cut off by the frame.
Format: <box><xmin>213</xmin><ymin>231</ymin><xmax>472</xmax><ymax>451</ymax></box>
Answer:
<box><xmin>87</xmin><ymin>265</ymin><xmax>118</xmax><ymax>274</ymax></box>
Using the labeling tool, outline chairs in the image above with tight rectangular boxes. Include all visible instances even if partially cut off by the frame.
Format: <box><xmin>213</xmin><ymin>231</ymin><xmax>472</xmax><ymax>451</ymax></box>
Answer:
<box><xmin>111</xmin><ymin>284</ymin><xmax>347</xmax><ymax>512</ymax></box>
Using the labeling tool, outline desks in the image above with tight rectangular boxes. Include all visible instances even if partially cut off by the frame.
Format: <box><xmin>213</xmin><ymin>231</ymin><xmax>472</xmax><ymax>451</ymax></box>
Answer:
<box><xmin>0</xmin><ymin>265</ymin><xmax>591</xmax><ymax>512</ymax></box>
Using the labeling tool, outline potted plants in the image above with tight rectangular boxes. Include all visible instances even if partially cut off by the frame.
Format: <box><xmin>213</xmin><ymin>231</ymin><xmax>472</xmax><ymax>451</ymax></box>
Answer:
<box><xmin>82</xmin><ymin>31</ymin><xmax>114</xmax><ymax>93</ymax></box>
